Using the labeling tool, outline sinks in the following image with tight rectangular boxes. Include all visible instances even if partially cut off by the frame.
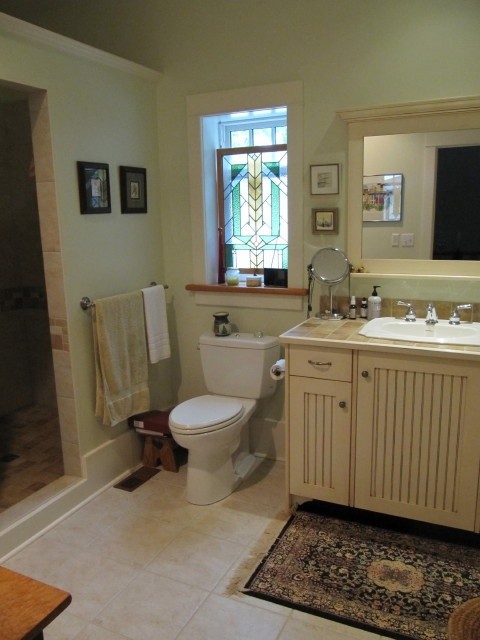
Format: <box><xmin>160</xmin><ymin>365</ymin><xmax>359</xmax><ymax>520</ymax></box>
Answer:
<box><xmin>358</xmin><ymin>316</ymin><xmax>480</xmax><ymax>346</ymax></box>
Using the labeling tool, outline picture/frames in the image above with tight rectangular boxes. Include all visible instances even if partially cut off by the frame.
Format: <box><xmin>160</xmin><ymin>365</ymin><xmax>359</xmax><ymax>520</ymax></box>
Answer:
<box><xmin>309</xmin><ymin>163</ymin><xmax>339</xmax><ymax>195</ymax></box>
<box><xmin>311</xmin><ymin>207</ymin><xmax>338</xmax><ymax>236</ymax></box>
<box><xmin>77</xmin><ymin>161</ymin><xmax>111</xmax><ymax>214</ymax></box>
<box><xmin>363</xmin><ymin>173</ymin><xmax>402</xmax><ymax>222</ymax></box>
<box><xmin>119</xmin><ymin>165</ymin><xmax>148</xmax><ymax>213</ymax></box>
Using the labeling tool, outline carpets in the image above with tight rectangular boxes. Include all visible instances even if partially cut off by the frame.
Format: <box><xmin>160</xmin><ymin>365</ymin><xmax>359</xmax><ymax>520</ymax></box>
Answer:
<box><xmin>223</xmin><ymin>507</ymin><xmax>480</xmax><ymax>640</ymax></box>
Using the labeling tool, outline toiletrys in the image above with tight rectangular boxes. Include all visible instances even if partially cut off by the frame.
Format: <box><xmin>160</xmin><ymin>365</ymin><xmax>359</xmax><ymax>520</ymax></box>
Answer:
<box><xmin>349</xmin><ymin>296</ymin><xmax>357</xmax><ymax>319</ymax></box>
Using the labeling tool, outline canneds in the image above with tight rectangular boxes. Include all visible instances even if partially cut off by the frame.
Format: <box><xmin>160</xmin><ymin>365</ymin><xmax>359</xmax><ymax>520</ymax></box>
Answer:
<box><xmin>225</xmin><ymin>267</ymin><xmax>240</xmax><ymax>286</ymax></box>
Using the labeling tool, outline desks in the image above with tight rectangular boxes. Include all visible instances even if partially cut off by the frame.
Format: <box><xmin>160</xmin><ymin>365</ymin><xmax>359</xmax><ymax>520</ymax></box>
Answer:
<box><xmin>0</xmin><ymin>563</ymin><xmax>72</xmax><ymax>640</ymax></box>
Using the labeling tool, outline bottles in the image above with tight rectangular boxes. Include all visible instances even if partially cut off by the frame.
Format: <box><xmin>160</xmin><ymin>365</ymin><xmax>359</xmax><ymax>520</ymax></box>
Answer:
<box><xmin>245</xmin><ymin>276</ymin><xmax>261</xmax><ymax>286</ymax></box>
<box><xmin>349</xmin><ymin>296</ymin><xmax>356</xmax><ymax>319</ymax></box>
<box><xmin>226</xmin><ymin>269</ymin><xmax>239</xmax><ymax>285</ymax></box>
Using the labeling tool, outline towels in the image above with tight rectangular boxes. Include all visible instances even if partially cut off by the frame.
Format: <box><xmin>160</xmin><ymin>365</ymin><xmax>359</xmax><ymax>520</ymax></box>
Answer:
<box><xmin>92</xmin><ymin>290</ymin><xmax>150</xmax><ymax>426</ymax></box>
<box><xmin>141</xmin><ymin>284</ymin><xmax>171</xmax><ymax>364</ymax></box>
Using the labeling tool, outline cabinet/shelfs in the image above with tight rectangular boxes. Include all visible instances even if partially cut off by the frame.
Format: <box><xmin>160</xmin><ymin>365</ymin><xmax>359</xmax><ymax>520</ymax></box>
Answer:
<box><xmin>354</xmin><ymin>351</ymin><xmax>479</xmax><ymax>532</ymax></box>
<box><xmin>288</xmin><ymin>343</ymin><xmax>353</xmax><ymax>509</ymax></box>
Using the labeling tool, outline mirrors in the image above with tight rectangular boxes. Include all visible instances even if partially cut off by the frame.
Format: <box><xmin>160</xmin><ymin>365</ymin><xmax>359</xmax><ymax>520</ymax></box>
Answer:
<box><xmin>361</xmin><ymin>127</ymin><xmax>479</xmax><ymax>262</ymax></box>
<box><xmin>310</xmin><ymin>247</ymin><xmax>349</xmax><ymax>281</ymax></box>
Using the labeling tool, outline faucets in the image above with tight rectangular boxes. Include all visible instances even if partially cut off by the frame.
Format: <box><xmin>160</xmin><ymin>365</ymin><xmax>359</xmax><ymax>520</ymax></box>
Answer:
<box><xmin>426</xmin><ymin>302</ymin><xmax>438</xmax><ymax>325</ymax></box>
